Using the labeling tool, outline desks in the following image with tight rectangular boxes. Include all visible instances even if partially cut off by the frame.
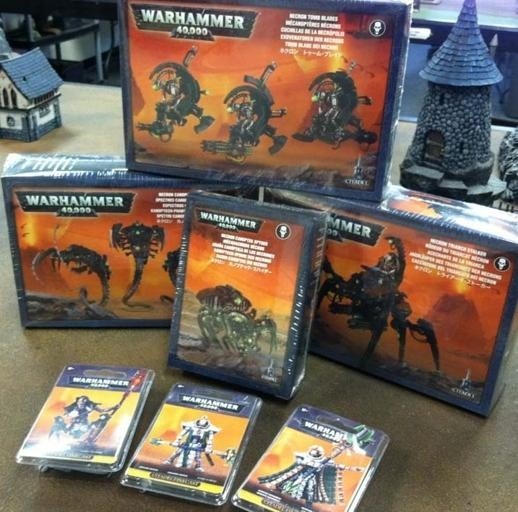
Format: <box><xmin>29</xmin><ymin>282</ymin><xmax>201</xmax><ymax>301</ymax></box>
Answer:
<box><xmin>0</xmin><ymin>81</ymin><xmax>518</xmax><ymax>512</ymax></box>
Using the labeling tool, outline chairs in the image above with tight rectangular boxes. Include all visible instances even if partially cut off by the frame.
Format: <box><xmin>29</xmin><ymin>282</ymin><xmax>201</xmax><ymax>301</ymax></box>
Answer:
<box><xmin>0</xmin><ymin>0</ymin><xmax>105</xmax><ymax>83</ymax></box>
